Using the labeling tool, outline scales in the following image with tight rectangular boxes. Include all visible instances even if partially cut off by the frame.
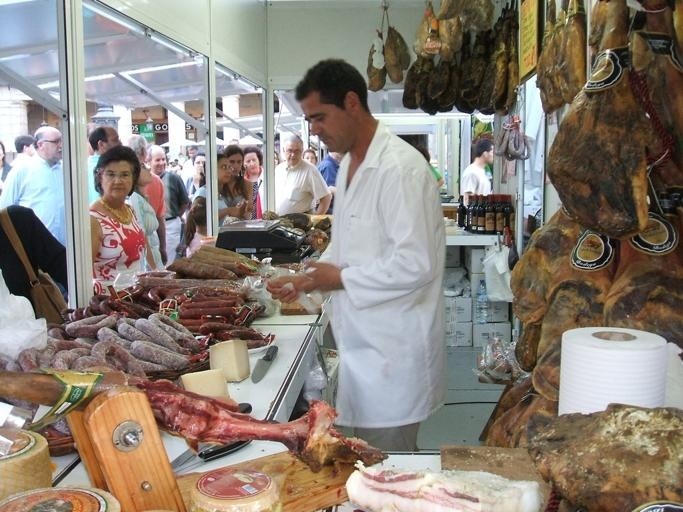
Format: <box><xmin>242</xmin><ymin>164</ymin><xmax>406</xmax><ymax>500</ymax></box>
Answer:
<box><xmin>215</xmin><ymin>219</ymin><xmax>307</xmax><ymax>255</ymax></box>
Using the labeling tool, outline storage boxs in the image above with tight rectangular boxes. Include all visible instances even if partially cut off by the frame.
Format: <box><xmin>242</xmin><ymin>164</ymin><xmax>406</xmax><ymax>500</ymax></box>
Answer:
<box><xmin>444</xmin><ymin>245</ymin><xmax>512</xmax><ymax>350</ymax></box>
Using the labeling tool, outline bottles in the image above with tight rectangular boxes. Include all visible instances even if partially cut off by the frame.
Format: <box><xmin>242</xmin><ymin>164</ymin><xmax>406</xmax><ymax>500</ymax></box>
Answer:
<box><xmin>459</xmin><ymin>193</ymin><xmax>514</xmax><ymax>235</ymax></box>
<box><xmin>476</xmin><ymin>282</ymin><xmax>493</xmax><ymax>321</ymax></box>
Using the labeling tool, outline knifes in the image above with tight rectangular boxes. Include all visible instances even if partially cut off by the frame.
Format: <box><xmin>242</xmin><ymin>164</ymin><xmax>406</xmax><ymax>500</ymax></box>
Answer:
<box><xmin>168</xmin><ymin>401</ymin><xmax>254</xmax><ymax>471</ymax></box>
<box><xmin>250</xmin><ymin>342</ymin><xmax>279</xmax><ymax>387</ymax></box>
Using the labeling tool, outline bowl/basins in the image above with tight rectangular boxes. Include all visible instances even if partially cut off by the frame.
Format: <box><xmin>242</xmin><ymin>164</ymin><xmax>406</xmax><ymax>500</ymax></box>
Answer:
<box><xmin>440</xmin><ymin>194</ymin><xmax>455</xmax><ymax>202</ymax></box>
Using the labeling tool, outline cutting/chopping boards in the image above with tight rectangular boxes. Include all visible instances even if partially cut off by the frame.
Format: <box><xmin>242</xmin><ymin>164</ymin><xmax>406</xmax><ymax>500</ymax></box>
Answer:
<box><xmin>440</xmin><ymin>443</ymin><xmax>547</xmax><ymax>510</ymax></box>
<box><xmin>177</xmin><ymin>439</ymin><xmax>361</xmax><ymax>512</ymax></box>
<box><xmin>280</xmin><ymin>296</ymin><xmax>323</xmax><ymax>316</ymax></box>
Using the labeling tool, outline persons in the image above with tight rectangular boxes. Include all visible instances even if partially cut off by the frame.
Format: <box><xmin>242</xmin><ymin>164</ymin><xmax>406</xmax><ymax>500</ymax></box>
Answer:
<box><xmin>415</xmin><ymin>144</ymin><xmax>444</xmax><ymax>188</ymax></box>
<box><xmin>264</xmin><ymin>57</ymin><xmax>450</xmax><ymax>452</ymax></box>
<box><xmin>458</xmin><ymin>140</ymin><xmax>494</xmax><ymax>209</ymax></box>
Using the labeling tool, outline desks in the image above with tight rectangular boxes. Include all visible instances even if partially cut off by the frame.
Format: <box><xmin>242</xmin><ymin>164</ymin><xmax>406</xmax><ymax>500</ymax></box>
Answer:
<box><xmin>50</xmin><ymin>226</ymin><xmax>441</xmax><ymax>511</ymax></box>
<box><xmin>440</xmin><ymin>218</ymin><xmax>505</xmax><ymax>248</ymax></box>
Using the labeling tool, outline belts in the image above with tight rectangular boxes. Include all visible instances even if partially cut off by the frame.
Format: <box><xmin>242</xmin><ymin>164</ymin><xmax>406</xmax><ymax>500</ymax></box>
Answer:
<box><xmin>163</xmin><ymin>216</ymin><xmax>178</xmax><ymax>221</ymax></box>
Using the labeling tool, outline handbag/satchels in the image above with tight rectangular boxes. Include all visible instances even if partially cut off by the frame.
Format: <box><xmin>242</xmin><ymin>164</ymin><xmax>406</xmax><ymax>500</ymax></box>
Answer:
<box><xmin>0</xmin><ymin>205</ymin><xmax>67</xmax><ymax>326</ymax></box>
